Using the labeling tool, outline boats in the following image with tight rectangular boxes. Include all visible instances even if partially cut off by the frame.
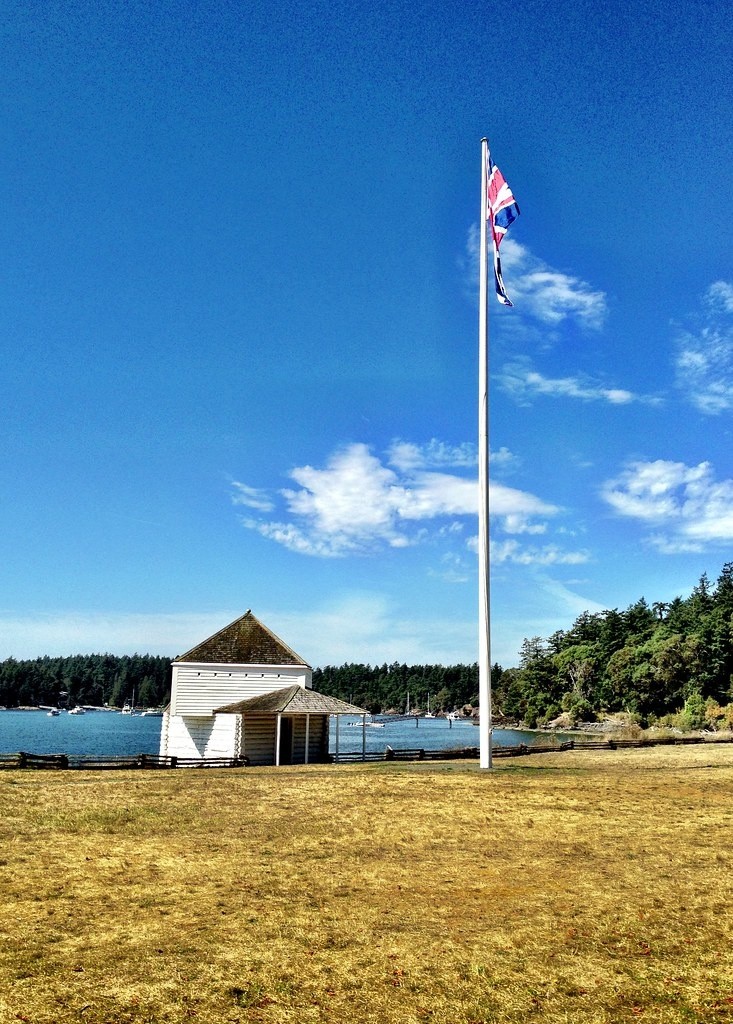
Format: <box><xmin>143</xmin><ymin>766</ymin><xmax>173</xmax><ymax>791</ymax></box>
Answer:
<box><xmin>446</xmin><ymin>713</ymin><xmax>462</xmax><ymax>722</ymax></box>
<box><xmin>139</xmin><ymin>708</ymin><xmax>163</xmax><ymax>717</ymax></box>
<box><xmin>424</xmin><ymin>712</ymin><xmax>435</xmax><ymax>719</ymax></box>
<box><xmin>67</xmin><ymin>705</ymin><xmax>86</xmax><ymax>715</ymax></box>
<box><xmin>47</xmin><ymin>709</ymin><xmax>61</xmax><ymax>717</ymax></box>
<box><xmin>121</xmin><ymin>704</ymin><xmax>135</xmax><ymax>715</ymax></box>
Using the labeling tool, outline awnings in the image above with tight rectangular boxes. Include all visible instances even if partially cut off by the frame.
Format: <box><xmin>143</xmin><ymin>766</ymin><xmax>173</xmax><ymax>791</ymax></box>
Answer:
<box><xmin>213</xmin><ymin>684</ymin><xmax>370</xmax><ymax>765</ymax></box>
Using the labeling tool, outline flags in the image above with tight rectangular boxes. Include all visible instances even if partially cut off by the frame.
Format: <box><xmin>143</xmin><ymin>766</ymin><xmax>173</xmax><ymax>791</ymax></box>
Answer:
<box><xmin>486</xmin><ymin>148</ymin><xmax>520</xmax><ymax>307</ymax></box>
